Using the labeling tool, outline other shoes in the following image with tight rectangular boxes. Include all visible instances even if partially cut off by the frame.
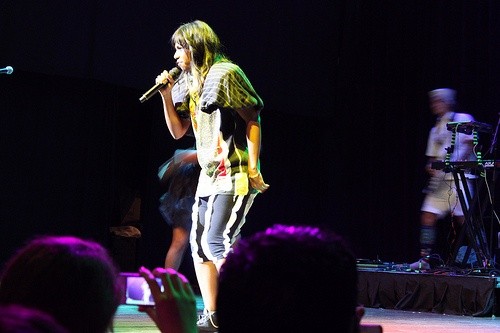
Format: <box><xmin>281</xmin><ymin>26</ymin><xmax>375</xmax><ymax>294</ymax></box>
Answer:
<box><xmin>196</xmin><ymin>309</ymin><xmax>224</xmax><ymax>333</ymax></box>
<box><xmin>409</xmin><ymin>262</ymin><xmax>432</xmax><ymax>272</ymax></box>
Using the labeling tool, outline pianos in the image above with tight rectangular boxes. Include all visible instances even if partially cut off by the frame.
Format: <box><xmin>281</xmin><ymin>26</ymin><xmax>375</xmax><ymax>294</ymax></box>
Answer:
<box><xmin>432</xmin><ymin>157</ymin><xmax>500</xmax><ymax>172</ymax></box>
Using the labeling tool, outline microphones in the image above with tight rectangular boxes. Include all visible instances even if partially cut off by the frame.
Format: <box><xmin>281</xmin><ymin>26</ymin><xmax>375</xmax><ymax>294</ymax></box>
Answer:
<box><xmin>139</xmin><ymin>67</ymin><xmax>180</xmax><ymax>103</ymax></box>
<box><xmin>0</xmin><ymin>66</ymin><xmax>13</xmax><ymax>74</ymax></box>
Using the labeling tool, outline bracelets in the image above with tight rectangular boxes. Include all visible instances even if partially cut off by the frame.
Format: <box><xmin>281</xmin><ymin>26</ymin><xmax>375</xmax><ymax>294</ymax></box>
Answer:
<box><xmin>249</xmin><ymin>171</ymin><xmax>261</xmax><ymax>179</ymax></box>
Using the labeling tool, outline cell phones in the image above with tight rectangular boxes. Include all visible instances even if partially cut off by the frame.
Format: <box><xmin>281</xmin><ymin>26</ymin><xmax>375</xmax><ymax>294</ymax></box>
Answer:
<box><xmin>123</xmin><ymin>273</ymin><xmax>165</xmax><ymax>306</ymax></box>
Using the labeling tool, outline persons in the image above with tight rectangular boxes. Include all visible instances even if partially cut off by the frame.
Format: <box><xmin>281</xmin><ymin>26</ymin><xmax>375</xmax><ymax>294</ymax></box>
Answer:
<box><xmin>407</xmin><ymin>88</ymin><xmax>477</xmax><ymax>271</ymax></box>
<box><xmin>155</xmin><ymin>21</ymin><xmax>269</xmax><ymax>333</ymax></box>
<box><xmin>0</xmin><ymin>145</ymin><xmax>368</xmax><ymax>333</ymax></box>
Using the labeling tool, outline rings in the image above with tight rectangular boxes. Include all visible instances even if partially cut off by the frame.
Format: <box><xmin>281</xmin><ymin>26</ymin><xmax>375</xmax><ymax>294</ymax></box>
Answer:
<box><xmin>260</xmin><ymin>186</ymin><xmax>264</xmax><ymax>189</ymax></box>
